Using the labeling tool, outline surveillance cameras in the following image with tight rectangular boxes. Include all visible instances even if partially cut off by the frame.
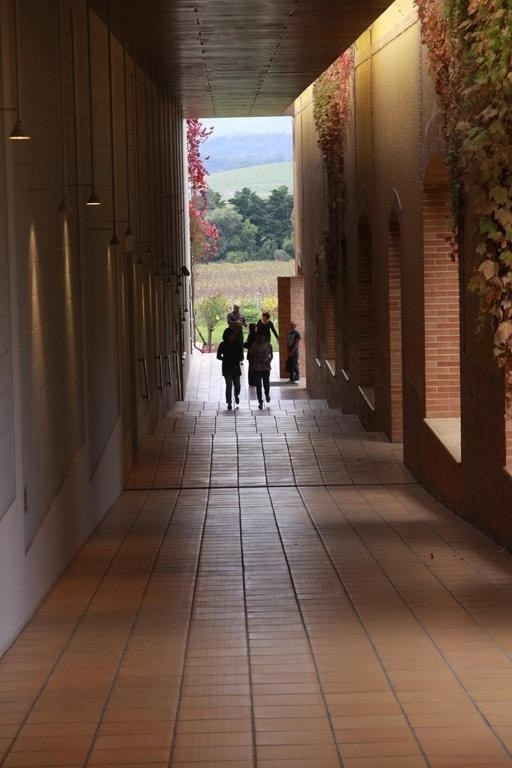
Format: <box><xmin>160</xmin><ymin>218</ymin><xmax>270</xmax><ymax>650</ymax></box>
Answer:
<box><xmin>176</xmin><ymin>266</ymin><xmax>190</xmax><ymax>285</ymax></box>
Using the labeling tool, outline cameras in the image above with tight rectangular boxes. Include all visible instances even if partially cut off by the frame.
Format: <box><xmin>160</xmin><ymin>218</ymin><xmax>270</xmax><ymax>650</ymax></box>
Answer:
<box><xmin>240</xmin><ymin>317</ymin><xmax>247</xmax><ymax>328</ymax></box>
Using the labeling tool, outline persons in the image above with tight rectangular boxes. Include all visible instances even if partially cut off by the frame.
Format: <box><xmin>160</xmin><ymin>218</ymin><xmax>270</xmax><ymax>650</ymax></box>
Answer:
<box><xmin>217</xmin><ymin>303</ymin><xmax>302</xmax><ymax>410</ymax></box>
